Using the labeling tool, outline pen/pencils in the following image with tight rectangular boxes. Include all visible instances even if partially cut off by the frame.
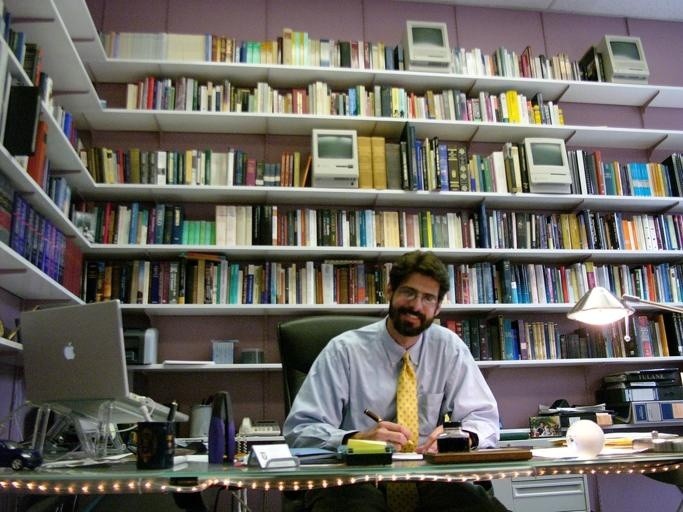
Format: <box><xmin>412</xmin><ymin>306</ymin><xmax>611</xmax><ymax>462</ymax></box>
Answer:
<box><xmin>167</xmin><ymin>401</ymin><xmax>177</xmax><ymax>421</ymax></box>
<box><xmin>201</xmin><ymin>394</ymin><xmax>215</xmax><ymax>406</ymax></box>
<box><xmin>140</xmin><ymin>407</ymin><xmax>153</xmax><ymax>422</ymax></box>
<box><xmin>363</xmin><ymin>409</ymin><xmax>415</xmax><ymax>445</ymax></box>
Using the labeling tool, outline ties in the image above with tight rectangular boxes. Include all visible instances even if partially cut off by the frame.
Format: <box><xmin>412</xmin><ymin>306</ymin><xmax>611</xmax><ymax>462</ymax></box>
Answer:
<box><xmin>394</xmin><ymin>351</ymin><xmax>421</xmax><ymax>452</ymax></box>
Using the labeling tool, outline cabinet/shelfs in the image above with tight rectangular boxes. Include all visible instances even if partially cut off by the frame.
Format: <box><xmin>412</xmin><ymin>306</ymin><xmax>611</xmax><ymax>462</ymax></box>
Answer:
<box><xmin>0</xmin><ymin>0</ymin><xmax>683</xmax><ymax>375</ymax></box>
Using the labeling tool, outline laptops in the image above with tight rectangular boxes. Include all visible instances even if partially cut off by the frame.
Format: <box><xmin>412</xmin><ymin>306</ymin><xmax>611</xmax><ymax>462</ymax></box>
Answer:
<box><xmin>20</xmin><ymin>300</ymin><xmax>189</xmax><ymax>425</ymax></box>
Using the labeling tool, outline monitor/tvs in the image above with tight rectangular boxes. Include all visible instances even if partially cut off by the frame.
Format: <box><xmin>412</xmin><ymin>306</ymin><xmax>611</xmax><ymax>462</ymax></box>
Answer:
<box><xmin>403</xmin><ymin>20</ymin><xmax>453</xmax><ymax>74</ymax></box>
<box><xmin>522</xmin><ymin>137</ymin><xmax>573</xmax><ymax>194</ymax></box>
<box><xmin>596</xmin><ymin>33</ymin><xmax>650</xmax><ymax>85</ymax></box>
<box><xmin>311</xmin><ymin>127</ymin><xmax>360</xmax><ymax>189</ymax></box>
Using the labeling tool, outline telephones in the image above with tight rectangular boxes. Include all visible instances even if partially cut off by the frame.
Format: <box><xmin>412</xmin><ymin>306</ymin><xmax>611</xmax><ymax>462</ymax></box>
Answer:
<box><xmin>239</xmin><ymin>416</ymin><xmax>281</xmax><ymax>436</ymax></box>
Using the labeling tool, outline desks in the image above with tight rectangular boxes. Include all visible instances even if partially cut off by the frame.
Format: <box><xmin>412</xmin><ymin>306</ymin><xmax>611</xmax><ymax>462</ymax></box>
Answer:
<box><xmin>0</xmin><ymin>445</ymin><xmax>683</xmax><ymax>512</ymax></box>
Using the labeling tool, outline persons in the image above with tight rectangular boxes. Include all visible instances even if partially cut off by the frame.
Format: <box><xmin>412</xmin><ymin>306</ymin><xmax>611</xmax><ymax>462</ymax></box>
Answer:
<box><xmin>533</xmin><ymin>421</ymin><xmax>558</xmax><ymax>435</ymax></box>
<box><xmin>279</xmin><ymin>250</ymin><xmax>515</xmax><ymax>511</ymax></box>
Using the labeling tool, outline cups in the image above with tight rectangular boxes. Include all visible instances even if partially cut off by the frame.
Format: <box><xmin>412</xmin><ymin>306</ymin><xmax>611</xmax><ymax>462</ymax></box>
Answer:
<box><xmin>125</xmin><ymin>421</ymin><xmax>175</xmax><ymax>470</ymax></box>
<box><xmin>188</xmin><ymin>403</ymin><xmax>214</xmax><ymax>438</ymax></box>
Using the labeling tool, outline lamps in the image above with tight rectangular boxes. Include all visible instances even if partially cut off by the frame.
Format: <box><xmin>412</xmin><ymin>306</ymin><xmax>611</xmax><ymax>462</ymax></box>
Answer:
<box><xmin>566</xmin><ymin>286</ymin><xmax>683</xmax><ymax>344</ymax></box>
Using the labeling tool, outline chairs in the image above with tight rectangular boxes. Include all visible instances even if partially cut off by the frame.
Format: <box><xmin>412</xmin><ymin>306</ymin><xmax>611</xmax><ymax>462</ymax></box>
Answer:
<box><xmin>276</xmin><ymin>315</ymin><xmax>494</xmax><ymax>497</ymax></box>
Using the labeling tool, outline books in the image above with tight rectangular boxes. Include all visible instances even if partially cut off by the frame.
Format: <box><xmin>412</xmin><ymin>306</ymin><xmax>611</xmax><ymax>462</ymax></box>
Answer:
<box><xmin>0</xmin><ymin>7</ymin><xmax>87</xmax><ymax>301</ymax></box>
<box><xmin>86</xmin><ymin>201</ymin><xmax>682</xmax><ymax>307</ymax></box>
<box><xmin>125</xmin><ymin>75</ymin><xmax>564</xmax><ymax>127</ymax></box>
<box><xmin>600</xmin><ymin>366</ymin><xmax>682</xmax><ymax>390</ymax></box>
<box><xmin>430</xmin><ymin>307</ymin><xmax>683</xmax><ymax>363</ymax></box>
<box><xmin>86</xmin><ymin>121</ymin><xmax>683</xmax><ymax>197</ymax></box>
<box><xmin>97</xmin><ymin>31</ymin><xmax>606</xmax><ymax>83</ymax></box>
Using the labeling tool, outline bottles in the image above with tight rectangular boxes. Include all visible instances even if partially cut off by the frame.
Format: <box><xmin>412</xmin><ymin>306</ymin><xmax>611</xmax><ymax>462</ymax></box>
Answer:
<box><xmin>437</xmin><ymin>420</ymin><xmax>470</xmax><ymax>453</ymax></box>
